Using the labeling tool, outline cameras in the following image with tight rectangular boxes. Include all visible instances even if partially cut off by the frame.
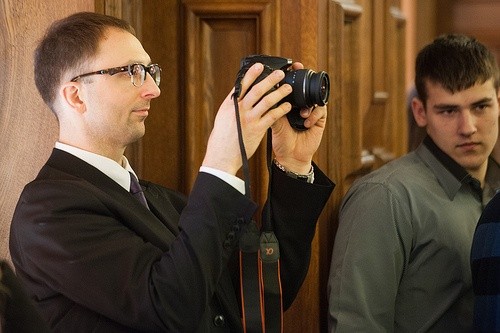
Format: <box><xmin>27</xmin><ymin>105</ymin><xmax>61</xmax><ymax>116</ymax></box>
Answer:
<box><xmin>241</xmin><ymin>55</ymin><xmax>330</xmax><ymax>131</ymax></box>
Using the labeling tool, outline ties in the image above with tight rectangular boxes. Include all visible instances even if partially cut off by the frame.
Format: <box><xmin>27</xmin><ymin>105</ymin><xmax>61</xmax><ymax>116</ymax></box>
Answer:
<box><xmin>129</xmin><ymin>171</ymin><xmax>146</xmax><ymax>208</ymax></box>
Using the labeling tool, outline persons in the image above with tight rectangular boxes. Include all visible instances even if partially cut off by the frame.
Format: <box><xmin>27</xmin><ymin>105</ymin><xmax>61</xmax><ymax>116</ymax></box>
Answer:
<box><xmin>9</xmin><ymin>12</ymin><xmax>336</xmax><ymax>333</ymax></box>
<box><xmin>328</xmin><ymin>30</ymin><xmax>500</xmax><ymax>332</ymax></box>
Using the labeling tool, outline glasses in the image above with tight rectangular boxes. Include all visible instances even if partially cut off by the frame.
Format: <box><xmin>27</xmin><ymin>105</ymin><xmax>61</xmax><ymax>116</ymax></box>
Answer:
<box><xmin>70</xmin><ymin>62</ymin><xmax>161</xmax><ymax>87</ymax></box>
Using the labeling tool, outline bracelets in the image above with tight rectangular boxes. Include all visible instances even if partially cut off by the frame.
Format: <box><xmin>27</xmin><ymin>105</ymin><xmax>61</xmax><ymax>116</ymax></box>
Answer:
<box><xmin>272</xmin><ymin>159</ymin><xmax>316</xmax><ymax>183</ymax></box>
